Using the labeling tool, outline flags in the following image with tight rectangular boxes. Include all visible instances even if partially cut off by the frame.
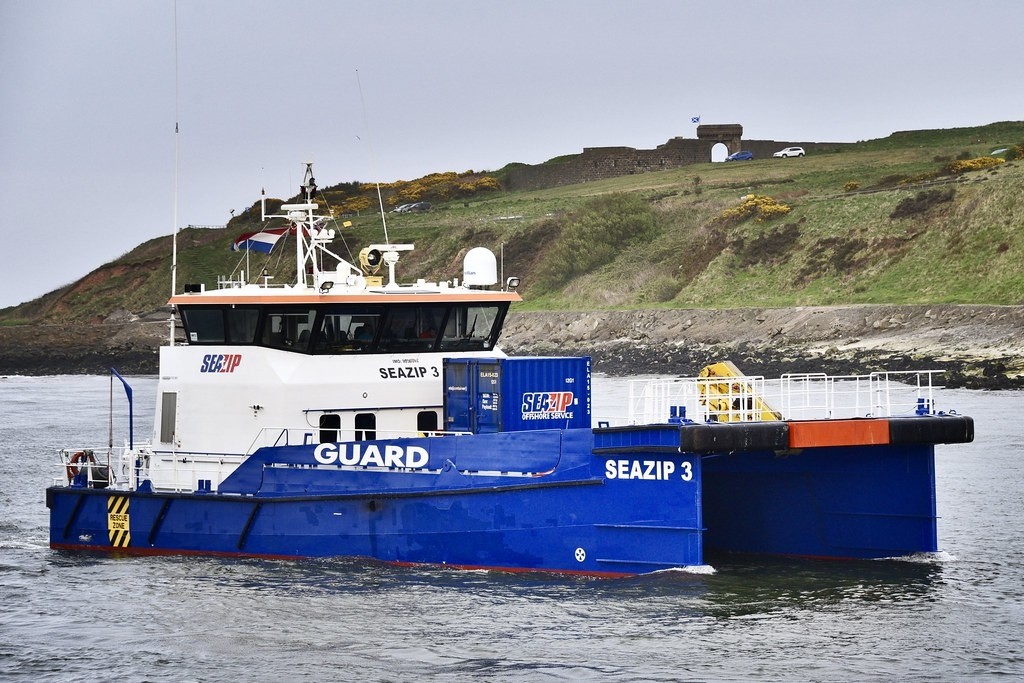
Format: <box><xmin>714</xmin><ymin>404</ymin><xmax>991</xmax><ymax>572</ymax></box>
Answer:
<box><xmin>691</xmin><ymin>116</ymin><xmax>698</xmax><ymax>123</ymax></box>
<box><xmin>229</xmin><ymin>226</ymin><xmax>289</xmax><ymax>254</ymax></box>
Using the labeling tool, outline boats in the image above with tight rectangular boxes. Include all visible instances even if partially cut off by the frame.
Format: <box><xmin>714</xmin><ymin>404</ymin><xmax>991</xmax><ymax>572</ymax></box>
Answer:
<box><xmin>45</xmin><ymin>120</ymin><xmax>974</xmax><ymax>579</ymax></box>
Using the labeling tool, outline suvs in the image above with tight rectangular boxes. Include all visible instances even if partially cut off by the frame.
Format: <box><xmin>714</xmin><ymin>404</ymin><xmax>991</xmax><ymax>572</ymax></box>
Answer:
<box><xmin>773</xmin><ymin>147</ymin><xmax>805</xmax><ymax>159</ymax></box>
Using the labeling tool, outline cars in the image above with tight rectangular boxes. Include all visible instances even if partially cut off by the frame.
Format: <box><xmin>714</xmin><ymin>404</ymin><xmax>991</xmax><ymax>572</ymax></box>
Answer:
<box><xmin>725</xmin><ymin>151</ymin><xmax>753</xmax><ymax>162</ymax></box>
<box><xmin>393</xmin><ymin>203</ymin><xmax>431</xmax><ymax>213</ymax></box>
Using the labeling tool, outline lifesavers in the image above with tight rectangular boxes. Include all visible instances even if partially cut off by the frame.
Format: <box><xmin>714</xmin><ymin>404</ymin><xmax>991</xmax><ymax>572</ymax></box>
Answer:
<box><xmin>70</xmin><ymin>451</ymin><xmax>97</xmax><ymax>474</ymax></box>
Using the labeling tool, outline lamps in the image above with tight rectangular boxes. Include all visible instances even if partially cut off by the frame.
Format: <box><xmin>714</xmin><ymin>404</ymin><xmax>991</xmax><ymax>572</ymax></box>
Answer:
<box><xmin>506</xmin><ymin>276</ymin><xmax>519</xmax><ymax>291</ymax></box>
<box><xmin>262</xmin><ymin>267</ymin><xmax>268</xmax><ymax>275</ymax></box>
<box><xmin>318</xmin><ymin>281</ymin><xmax>333</xmax><ymax>292</ymax></box>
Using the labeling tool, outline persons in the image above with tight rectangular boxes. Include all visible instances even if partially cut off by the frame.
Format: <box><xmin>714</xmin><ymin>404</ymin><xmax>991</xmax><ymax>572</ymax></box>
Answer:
<box><xmin>420</xmin><ymin>327</ymin><xmax>438</xmax><ymax>339</ymax></box>
